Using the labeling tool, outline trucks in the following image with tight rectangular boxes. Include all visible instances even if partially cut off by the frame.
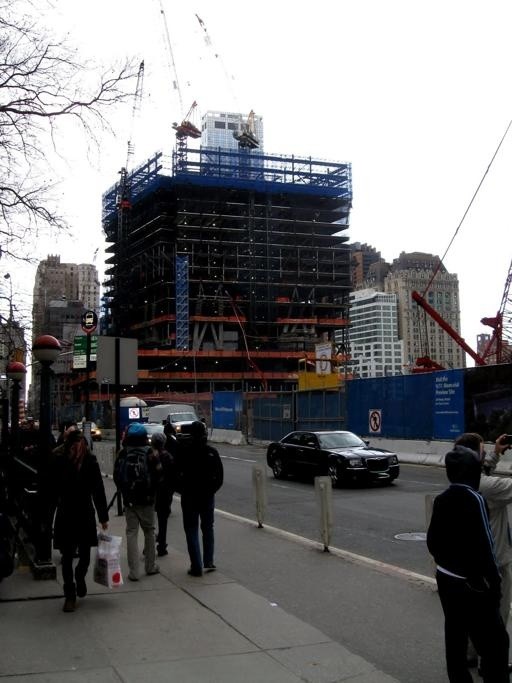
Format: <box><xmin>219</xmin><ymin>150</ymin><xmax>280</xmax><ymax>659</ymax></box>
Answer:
<box><xmin>149</xmin><ymin>402</ymin><xmax>209</xmax><ymax>443</ymax></box>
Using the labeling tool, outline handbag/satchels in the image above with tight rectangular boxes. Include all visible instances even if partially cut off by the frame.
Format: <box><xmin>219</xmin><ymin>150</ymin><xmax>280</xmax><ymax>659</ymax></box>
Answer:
<box><xmin>91</xmin><ymin>528</ymin><xmax>124</xmax><ymax>591</ymax></box>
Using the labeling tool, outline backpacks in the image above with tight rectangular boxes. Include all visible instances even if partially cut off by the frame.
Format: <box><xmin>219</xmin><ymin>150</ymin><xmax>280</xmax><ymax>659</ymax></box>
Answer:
<box><xmin>116</xmin><ymin>444</ymin><xmax>157</xmax><ymax>509</ymax></box>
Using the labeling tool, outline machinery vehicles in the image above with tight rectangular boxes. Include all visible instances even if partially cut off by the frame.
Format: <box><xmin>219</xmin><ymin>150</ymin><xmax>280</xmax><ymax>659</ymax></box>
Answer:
<box><xmin>233</xmin><ymin>108</ymin><xmax>260</xmax><ymax>149</ymax></box>
<box><xmin>113</xmin><ymin>57</ymin><xmax>146</xmax><ymax>213</ymax></box>
<box><xmin>171</xmin><ymin>99</ymin><xmax>202</xmax><ymax>139</ymax></box>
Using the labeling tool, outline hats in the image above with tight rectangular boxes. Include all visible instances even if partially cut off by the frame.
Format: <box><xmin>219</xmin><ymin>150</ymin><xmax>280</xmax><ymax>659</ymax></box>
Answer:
<box><xmin>189</xmin><ymin>421</ymin><xmax>204</xmax><ymax>435</ymax></box>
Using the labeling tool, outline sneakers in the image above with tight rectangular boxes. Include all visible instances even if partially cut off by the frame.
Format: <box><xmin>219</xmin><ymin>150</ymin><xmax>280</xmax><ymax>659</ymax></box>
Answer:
<box><xmin>188</xmin><ymin>570</ymin><xmax>203</xmax><ymax>578</ymax></box>
<box><xmin>203</xmin><ymin>564</ymin><xmax>217</xmax><ymax>573</ymax></box>
<box><xmin>145</xmin><ymin>565</ymin><xmax>161</xmax><ymax>576</ymax></box>
<box><xmin>128</xmin><ymin>574</ymin><xmax>140</xmax><ymax>583</ymax></box>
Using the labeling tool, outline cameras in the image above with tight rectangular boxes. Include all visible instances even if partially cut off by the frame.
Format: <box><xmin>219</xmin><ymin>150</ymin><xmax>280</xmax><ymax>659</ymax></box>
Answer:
<box><xmin>505</xmin><ymin>435</ymin><xmax>512</xmax><ymax>444</ymax></box>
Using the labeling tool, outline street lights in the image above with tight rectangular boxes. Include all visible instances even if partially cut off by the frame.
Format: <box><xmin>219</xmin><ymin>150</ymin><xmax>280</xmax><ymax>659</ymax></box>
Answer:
<box><xmin>4</xmin><ymin>271</ymin><xmax>12</xmax><ymax>403</ymax></box>
<box><xmin>32</xmin><ymin>332</ymin><xmax>62</xmax><ymax>432</ymax></box>
<box><xmin>7</xmin><ymin>361</ymin><xmax>26</xmax><ymax>428</ymax></box>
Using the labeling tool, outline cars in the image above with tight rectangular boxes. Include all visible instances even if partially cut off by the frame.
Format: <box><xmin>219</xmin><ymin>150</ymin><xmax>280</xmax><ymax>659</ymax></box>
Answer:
<box><xmin>267</xmin><ymin>427</ymin><xmax>400</xmax><ymax>486</ymax></box>
<box><xmin>76</xmin><ymin>418</ymin><xmax>102</xmax><ymax>441</ymax></box>
<box><xmin>141</xmin><ymin>423</ymin><xmax>165</xmax><ymax>446</ymax></box>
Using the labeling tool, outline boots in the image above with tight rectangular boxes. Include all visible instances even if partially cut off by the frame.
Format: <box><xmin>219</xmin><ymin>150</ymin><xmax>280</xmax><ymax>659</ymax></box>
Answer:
<box><xmin>62</xmin><ymin>582</ymin><xmax>77</xmax><ymax>612</ymax></box>
<box><xmin>75</xmin><ymin>565</ymin><xmax>88</xmax><ymax>598</ymax></box>
<box><xmin>156</xmin><ymin>542</ymin><xmax>168</xmax><ymax>556</ymax></box>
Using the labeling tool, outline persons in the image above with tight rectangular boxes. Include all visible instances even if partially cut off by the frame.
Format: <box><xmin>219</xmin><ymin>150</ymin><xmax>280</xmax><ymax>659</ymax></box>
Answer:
<box><xmin>19</xmin><ymin>415</ymin><xmax>107</xmax><ymax>612</ymax></box>
<box><xmin>456</xmin><ymin>433</ymin><xmax>512</xmax><ymax>676</ymax></box>
<box><xmin>113</xmin><ymin>417</ymin><xmax>223</xmax><ymax>580</ymax></box>
<box><xmin>425</xmin><ymin>445</ymin><xmax>510</xmax><ymax>683</ymax></box>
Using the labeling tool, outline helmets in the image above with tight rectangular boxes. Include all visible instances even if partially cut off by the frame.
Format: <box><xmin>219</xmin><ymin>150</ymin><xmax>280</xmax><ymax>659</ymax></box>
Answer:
<box><xmin>125</xmin><ymin>422</ymin><xmax>147</xmax><ymax>441</ymax></box>
<box><xmin>151</xmin><ymin>431</ymin><xmax>167</xmax><ymax>449</ymax></box>
